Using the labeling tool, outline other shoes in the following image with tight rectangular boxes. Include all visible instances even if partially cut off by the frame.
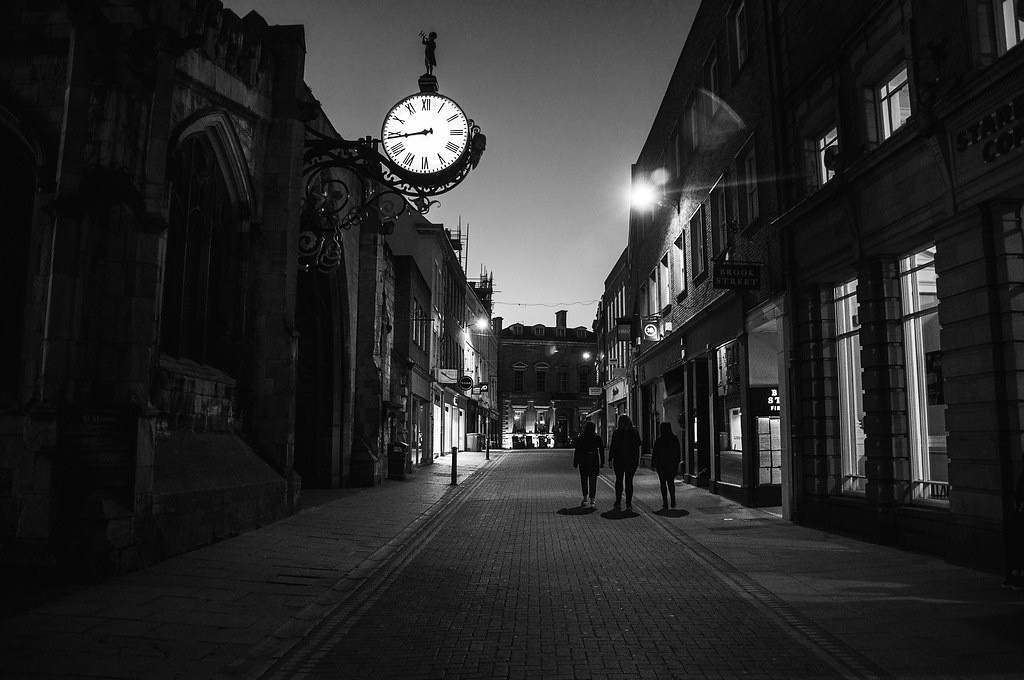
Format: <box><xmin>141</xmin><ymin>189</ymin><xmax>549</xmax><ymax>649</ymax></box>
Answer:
<box><xmin>613</xmin><ymin>507</ymin><xmax>622</xmax><ymax>514</ymax></box>
<box><xmin>590</xmin><ymin>499</ymin><xmax>595</xmax><ymax>506</ymax></box>
<box><xmin>623</xmin><ymin>509</ymin><xmax>632</xmax><ymax>516</ymax></box>
<box><xmin>582</xmin><ymin>499</ymin><xmax>588</xmax><ymax>506</ymax></box>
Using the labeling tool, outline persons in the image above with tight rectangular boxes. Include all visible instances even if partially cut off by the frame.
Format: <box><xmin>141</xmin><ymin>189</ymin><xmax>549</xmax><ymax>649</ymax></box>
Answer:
<box><xmin>573</xmin><ymin>420</ymin><xmax>605</xmax><ymax>509</ymax></box>
<box><xmin>651</xmin><ymin>422</ymin><xmax>681</xmax><ymax>510</ymax></box>
<box><xmin>608</xmin><ymin>413</ymin><xmax>641</xmax><ymax>512</ymax></box>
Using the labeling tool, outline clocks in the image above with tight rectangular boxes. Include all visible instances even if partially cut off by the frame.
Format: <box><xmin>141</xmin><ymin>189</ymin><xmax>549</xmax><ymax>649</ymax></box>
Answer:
<box><xmin>380</xmin><ymin>92</ymin><xmax>469</xmax><ymax>174</ymax></box>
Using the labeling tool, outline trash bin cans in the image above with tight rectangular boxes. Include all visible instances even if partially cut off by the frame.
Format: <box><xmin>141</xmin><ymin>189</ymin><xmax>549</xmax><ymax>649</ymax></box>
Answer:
<box><xmin>466</xmin><ymin>432</ymin><xmax>482</xmax><ymax>452</ymax></box>
<box><xmin>387</xmin><ymin>441</ymin><xmax>410</xmax><ymax>481</ymax></box>
